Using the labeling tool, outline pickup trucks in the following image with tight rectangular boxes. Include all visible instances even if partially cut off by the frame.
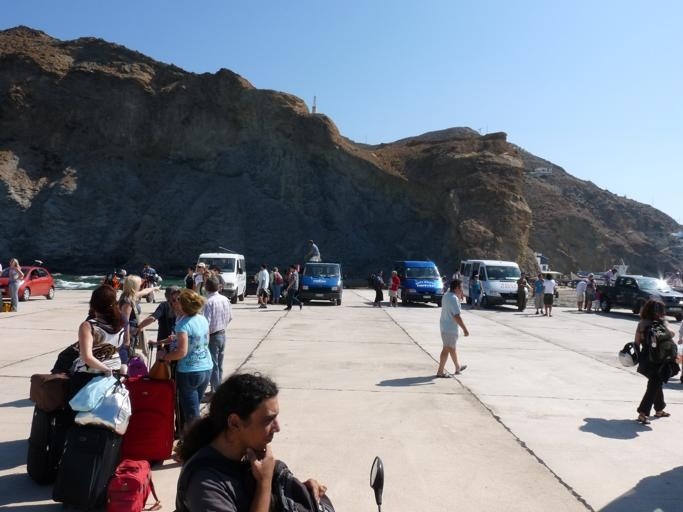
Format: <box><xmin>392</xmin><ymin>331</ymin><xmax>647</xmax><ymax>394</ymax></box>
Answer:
<box><xmin>593</xmin><ymin>275</ymin><xmax>683</xmax><ymax>322</ymax></box>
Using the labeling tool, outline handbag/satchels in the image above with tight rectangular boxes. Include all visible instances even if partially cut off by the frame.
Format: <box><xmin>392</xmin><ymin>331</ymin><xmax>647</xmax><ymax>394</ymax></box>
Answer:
<box><xmin>107</xmin><ymin>455</ymin><xmax>161</xmax><ymax>512</ymax></box>
<box><xmin>30</xmin><ymin>340</ymin><xmax>132</xmax><ymax>436</ymax></box>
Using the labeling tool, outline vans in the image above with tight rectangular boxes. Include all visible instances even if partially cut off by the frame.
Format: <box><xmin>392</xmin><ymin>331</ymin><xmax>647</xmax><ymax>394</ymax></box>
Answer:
<box><xmin>458</xmin><ymin>259</ymin><xmax>528</xmax><ymax>308</ymax></box>
<box><xmin>194</xmin><ymin>252</ymin><xmax>247</xmax><ymax>305</ymax></box>
<box><xmin>391</xmin><ymin>259</ymin><xmax>444</xmax><ymax>307</ymax></box>
<box><xmin>299</xmin><ymin>263</ymin><xmax>343</xmax><ymax>305</ymax></box>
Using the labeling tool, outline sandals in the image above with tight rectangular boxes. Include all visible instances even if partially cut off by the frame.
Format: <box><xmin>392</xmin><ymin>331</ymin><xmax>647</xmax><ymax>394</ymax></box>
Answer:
<box><xmin>653</xmin><ymin>412</ymin><xmax>669</xmax><ymax>417</ymax></box>
<box><xmin>636</xmin><ymin>415</ymin><xmax>649</xmax><ymax>424</ymax></box>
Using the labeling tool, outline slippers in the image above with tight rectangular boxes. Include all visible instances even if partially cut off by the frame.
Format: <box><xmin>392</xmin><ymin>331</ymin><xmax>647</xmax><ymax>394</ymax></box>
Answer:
<box><xmin>454</xmin><ymin>366</ymin><xmax>466</xmax><ymax>374</ymax></box>
<box><xmin>437</xmin><ymin>372</ymin><xmax>451</xmax><ymax>378</ymax></box>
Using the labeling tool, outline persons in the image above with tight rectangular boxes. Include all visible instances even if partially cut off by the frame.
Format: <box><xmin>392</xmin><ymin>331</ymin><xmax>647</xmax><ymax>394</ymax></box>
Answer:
<box><xmin>374</xmin><ymin>270</ymin><xmax>386</xmax><ymax>308</ymax></box>
<box><xmin>535</xmin><ymin>273</ymin><xmax>556</xmax><ymax>316</ymax></box>
<box><xmin>178</xmin><ymin>373</ymin><xmax>326</xmax><ymax>512</ymax></box>
<box><xmin>631</xmin><ymin>299</ymin><xmax>675</xmax><ymax>425</ymax></box>
<box><xmin>516</xmin><ymin>272</ymin><xmax>529</xmax><ymax>311</ymax></box>
<box><xmin>603</xmin><ymin>267</ymin><xmax>617</xmax><ymax>281</ymax></box>
<box><xmin>677</xmin><ymin>298</ymin><xmax>683</xmax><ymax>384</ymax></box>
<box><xmin>388</xmin><ymin>270</ymin><xmax>401</xmax><ymax>308</ymax></box>
<box><xmin>67</xmin><ymin>263</ymin><xmax>234</xmax><ymax>465</ymax></box>
<box><xmin>470</xmin><ymin>275</ymin><xmax>483</xmax><ymax>309</ymax></box>
<box><xmin>0</xmin><ymin>264</ymin><xmax>2</xmax><ymax>276</ymax></box>
<box><xmin>577</xmin><ymin>273</ymin><xmax>601</xmax><ymax>312</ymax></box>
<box><xmin>6</xmin><ymin>257</ymin><xmax>23</xmax><ymax>312</ymax></box>
<box><xmin>453</xmin><ymin>269</ymin><xmax>461</xmax><ymax>279</ymax></box>
<box><xmin>255</xmin><ymin>264</ymin><xmax>303</xmax><ymax>311</ymax></box>
<box><xmin>304</xmin><ymin>240</ymin><xmax>322</xmax><ymax>262</ymax></box>
<box><xmin>434</xmin><ymin>278</ymin><xmax>467</xmax><ymax>377</ymax></box>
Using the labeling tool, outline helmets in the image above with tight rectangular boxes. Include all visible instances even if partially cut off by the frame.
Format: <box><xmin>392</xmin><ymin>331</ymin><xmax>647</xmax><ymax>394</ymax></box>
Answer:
<box><xmin>618</xmin><ymin>342</ymin><xmax>640</xmax><ymax>367</ymax></box>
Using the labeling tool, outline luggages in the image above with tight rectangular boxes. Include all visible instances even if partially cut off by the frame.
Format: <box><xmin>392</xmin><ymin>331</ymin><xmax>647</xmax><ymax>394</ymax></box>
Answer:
<box><xmin>26</xmin><ymin>406</ymin><xmax>71</xmax><ymax>486</ymax></box>
<box><xmin>55</xmin><ymin>420</ymin><xmax>125</xmax><ymax>510</ymax></box>
<box><xmin>124</xmin><ymin>343</ymin><xmax>175</xmax><ymax>461</ymax></box>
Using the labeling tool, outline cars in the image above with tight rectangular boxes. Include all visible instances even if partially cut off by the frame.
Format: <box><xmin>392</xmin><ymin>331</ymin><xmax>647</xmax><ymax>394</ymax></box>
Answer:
<box><xmin>0</xmin><ymin>266</ymin><xmax>55</xmax><ymax>301</ymax></box>
<box><xmin>244</xmin><ymin>274</ymin><xmax>257</xmax><ymax>297</ymax></box>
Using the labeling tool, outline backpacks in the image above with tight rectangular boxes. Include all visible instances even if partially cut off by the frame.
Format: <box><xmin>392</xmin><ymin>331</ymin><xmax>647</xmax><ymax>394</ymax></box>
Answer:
<box><xmin>268</xmin><ymin>460</ymin><xmax>334</xmax><ymax>512</ymax></box>
<box><xmin>645</xmin><ymin>317</ymin><xmax>676</xmax><ymax>363</ymax></box>
